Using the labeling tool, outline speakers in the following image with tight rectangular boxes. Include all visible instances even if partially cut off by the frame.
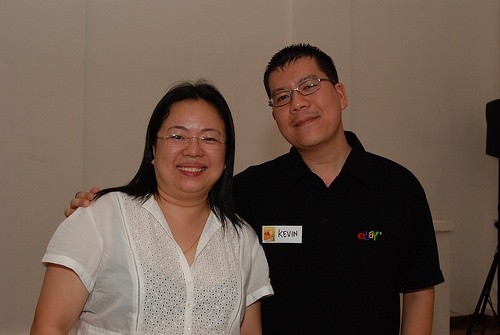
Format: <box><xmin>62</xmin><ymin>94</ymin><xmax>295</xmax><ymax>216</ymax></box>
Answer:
<box><xmin>485</xmin><ymin>99</ymin><xmax>500</xmax><ymax>157</ymax></box>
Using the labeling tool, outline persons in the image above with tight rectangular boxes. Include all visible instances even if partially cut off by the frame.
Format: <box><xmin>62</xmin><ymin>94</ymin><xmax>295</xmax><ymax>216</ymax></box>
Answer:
<box><xmin>63</xmin><ymin>44</ymin><xmax>446</xmax><ymax>335</ymax></box>
<box><xmin>30</xmin><ymin>77</ymin><xmax>274</xmax><ymax>335</ymax></box>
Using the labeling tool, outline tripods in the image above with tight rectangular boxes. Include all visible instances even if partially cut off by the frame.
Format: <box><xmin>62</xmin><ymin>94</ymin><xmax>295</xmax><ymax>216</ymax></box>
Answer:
<box><xmin>465</xmin><ymin>157</ymin><xmax>500</xmax><ymax>335</ymax></box>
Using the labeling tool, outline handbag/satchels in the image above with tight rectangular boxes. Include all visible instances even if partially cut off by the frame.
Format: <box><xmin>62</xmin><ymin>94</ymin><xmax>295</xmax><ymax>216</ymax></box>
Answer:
<box><xmin>449</xmin><ymin>252</ymin><xmax>500</xmax><ymax>335</ymax></box>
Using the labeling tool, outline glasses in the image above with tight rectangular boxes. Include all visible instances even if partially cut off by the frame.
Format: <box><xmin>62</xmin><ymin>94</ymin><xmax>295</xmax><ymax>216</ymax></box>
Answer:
<box><xmin>268</xmin><ymin>73</ymin><xmax>336</xmax><ymax>108</ymax></box>
<box><xmin>157</xmin><ymin>129</ymin><xmax>227</xmax><ymax>150</ymax></box>
